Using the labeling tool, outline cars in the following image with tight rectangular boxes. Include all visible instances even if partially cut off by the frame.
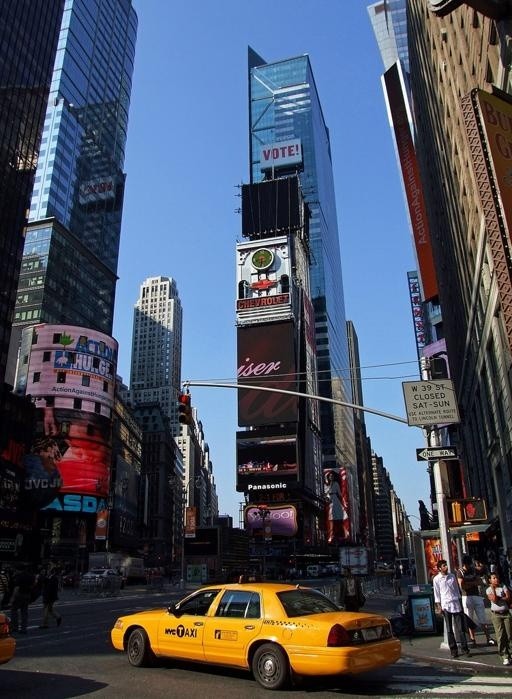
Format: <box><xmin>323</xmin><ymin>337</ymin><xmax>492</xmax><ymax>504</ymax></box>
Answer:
<box><xmin>36</xmin><ymin>568</ymin><xmax>126</xmax><ymax>590</ymax></box>
<box><xmin>288</xmin><ymin>554</ymin><xmax>416</xmax><ymax>579</ymax></box>
<box><xmin>110</xmin><ymin>581</ymin><xmax>401</xmax><ymax>690</ymax></box>
<box><xmin>0</xmin><ymin>611</ymin><xmax>17</xmax><ymax>666</ymax></box>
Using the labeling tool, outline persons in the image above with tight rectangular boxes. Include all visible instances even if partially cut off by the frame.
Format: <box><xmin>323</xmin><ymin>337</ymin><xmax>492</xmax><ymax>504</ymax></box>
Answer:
<box><xmin>0</xmin><ymin>558</ymin><xmax>85</xmax><ymax>634</ymax></box>
<box><xmin>44</xmin><ymin>405</ymin><xmax>59</xmax><ymax>438</ymax></box>
<box><xmin>431</xmin><ymin>553</ymin><xmax>511</xmax><ymax>667</ymax></box>
<box><xmin>411</xmin><ymin>558</ymin><xmax>416</xmax><ymax>583</ymax></box>
<box><xmin>392</xmin><ymin>561</ymin><xmax>402</xmax><ymax>597</ymax></box>
<box><xmin>337</xmin><ymin>567</ymin><xmax>366</xmax><ymax>613</ymax></box>
<box><xmin>321</xmin><ymin>470</ymin><xmax>349</xmax><ymax>542</ymax></box>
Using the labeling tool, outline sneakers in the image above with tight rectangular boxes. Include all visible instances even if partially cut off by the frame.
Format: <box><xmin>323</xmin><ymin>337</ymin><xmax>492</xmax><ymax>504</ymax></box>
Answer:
<box><xmin>503</xmin><ymin>658</ymin><xmax>510</xmax><ymax>665</ymax></box>
<box><xmin>467</xmin><ymin>638</ymin><xmax>475</xmax><ymax>645</ymax></box>
<box><xmin>451</xmin><ymin>649</ymin><xmax>459</xmax><ymax>657</ymax></box>
<box><xmin>488</xmin><ymin>637</ymin><xmax>496</xmax><ymax>645</ymax></box>
<box><xmin>461</xmin><ymin>648</ymin><xmax>470</xmax><ymax>655</ymax></box>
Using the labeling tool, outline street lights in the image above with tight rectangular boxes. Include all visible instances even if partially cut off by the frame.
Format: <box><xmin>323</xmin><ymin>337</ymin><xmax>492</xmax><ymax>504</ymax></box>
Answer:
<box><xmin>181</xmin><ymin>474</ymin><xmax>204</xmax><ymax>589</ymax></box>
<box><xmin>255</xmin><ymin>505</ymin><xmax>271</xmax><ymax>574</ymax></box>
<box><xmin>405</xmin><ymin>514</ymin><xmax>421</xmax><ymax>522</ymax></box>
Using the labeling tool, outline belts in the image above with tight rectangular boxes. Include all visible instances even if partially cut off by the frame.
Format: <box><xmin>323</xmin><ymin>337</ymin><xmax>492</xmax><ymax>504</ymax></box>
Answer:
<box><xmin>491</xmin><ymin>609</ymin><xmax>508</xmax><ymax>615</ymax></box>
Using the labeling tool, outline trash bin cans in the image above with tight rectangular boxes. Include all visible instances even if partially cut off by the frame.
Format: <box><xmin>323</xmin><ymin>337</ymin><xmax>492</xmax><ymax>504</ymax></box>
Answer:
<box><xmin>407</xmin><ymin>584</ymin><xmax>437</xmax><ymax>636</ymax></box>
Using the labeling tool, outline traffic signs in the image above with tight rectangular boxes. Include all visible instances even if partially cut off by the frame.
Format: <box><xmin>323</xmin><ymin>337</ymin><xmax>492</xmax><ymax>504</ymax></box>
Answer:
<box><xmin>401</xmin><ymin>378</ymin><xmax>461</xmax><ymax>426</ymax></box>
<box><xmin>415</xmin><ymin>447</ymin><xmax>458</xmax><ymax>462</ymax></box>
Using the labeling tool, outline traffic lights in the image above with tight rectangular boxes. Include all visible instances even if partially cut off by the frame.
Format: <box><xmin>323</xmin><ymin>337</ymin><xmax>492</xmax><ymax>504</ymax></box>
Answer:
<box><xmin>462</xmin><ymin>497</ymin><xmax>488</xmax><ymax>521</ymax></box>
<box><xmin>178</xmin><ymin>393</ymin><xmax>193</xmax><ymax>426</ymax></box>
<box><xmin>379</xmin><ymin>556</ymin><xmax>383</xmax><ymax>560</ymax></box>
<box><xmin>397</xmin><ymin>535</ymin><xmax>403</xmax><ymax>541</ymax></box>
